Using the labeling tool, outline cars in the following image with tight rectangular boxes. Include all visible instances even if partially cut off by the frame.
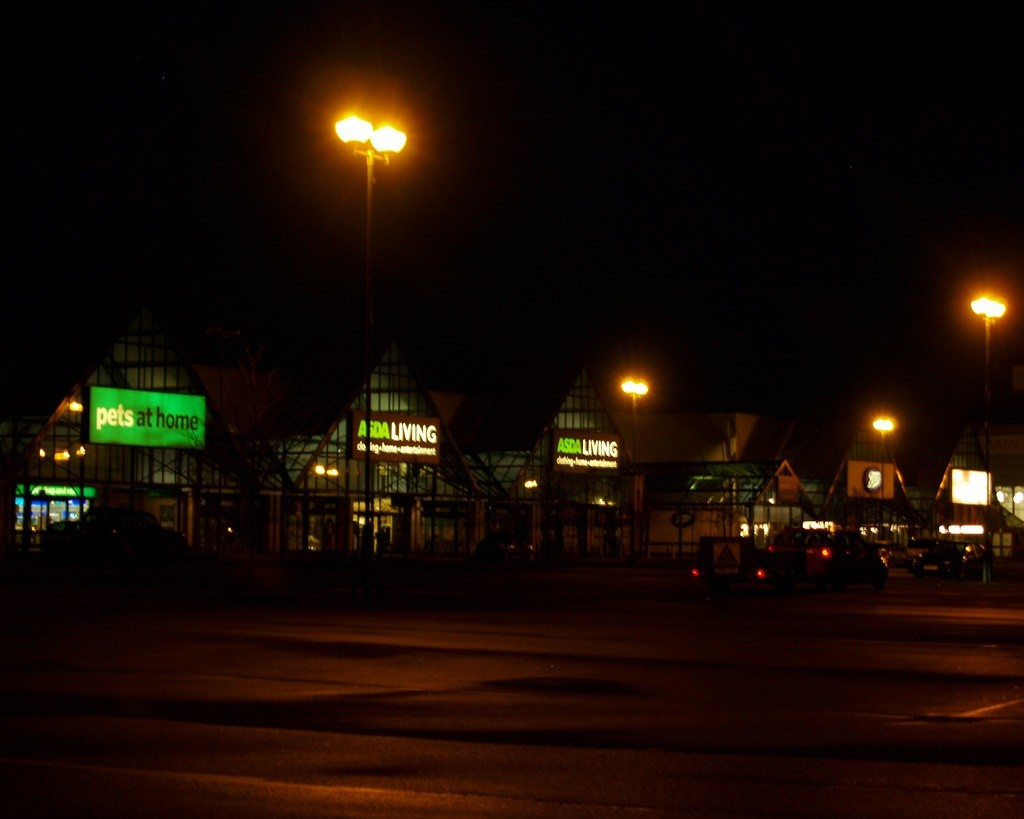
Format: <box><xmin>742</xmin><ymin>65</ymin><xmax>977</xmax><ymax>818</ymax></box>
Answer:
<box><xmin>869</xmin><ymin>541</ymin><xmax>915</xmax><ymax>572</ymax></box>
<box><xmin>951</xmin><ymin>542</ymin><xmax>985</xmax><ymax>574</ymax></box>
<box><xmin>475</xmin><ymin>532</ymin><xmax>538</xmax><ymax>562</ymax></box>
<box><xmin>36</xmin><ymin>505</ymin><xmax>188</xmax><ymax>576</ymax></box>
<box><xmin>767</xmin><ymin>527</ymin><xmax>887</xmax><ymax>591</ymax></box>
<box><xmin>913</xmin><ymin>544</ymin><xmax>969</xmax><ymax>582</ymax></box>
<box><xmin>907</xmin><ymin>541</ymin><xmax>955</xmax><ymax>560</ymax></box>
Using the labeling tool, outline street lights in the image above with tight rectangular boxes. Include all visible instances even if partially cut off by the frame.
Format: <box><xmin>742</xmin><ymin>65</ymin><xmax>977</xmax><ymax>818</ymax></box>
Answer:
<box><xmin>334</xmin><ymin>107</ymin><xmax>409</xmax><ymax>577</ymax></box>
<box><xmin>618</xmin><ymin>374</ymin><xmax>650</xmax><ymax>446</ymax></box>
<box><xmin>869</xmin><ymin>415</ymin><xmax>896</xmax><ymax>538</ymax></box>
<box><xmin>971</xmin><ymin>289</ymin><xmax>1010</xmax><ymax>587</ymax></box>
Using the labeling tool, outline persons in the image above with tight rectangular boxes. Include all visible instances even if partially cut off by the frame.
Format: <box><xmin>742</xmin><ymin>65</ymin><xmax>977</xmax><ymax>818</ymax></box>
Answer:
<box><xmin>797</xmin><ymin>532</ymin><xmax>818</xmax><ymax>544</ymax></box>
<box><xmin>361</xmin><ymin>522</ymin><xmax>390</xmax><ymax>560</ymax></box>
